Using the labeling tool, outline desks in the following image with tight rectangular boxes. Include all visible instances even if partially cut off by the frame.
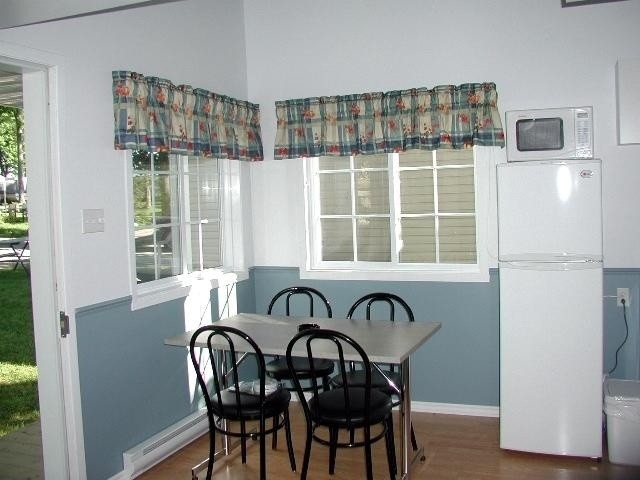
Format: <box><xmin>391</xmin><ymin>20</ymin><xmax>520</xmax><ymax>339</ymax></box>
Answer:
<box><xmin>163</xmin><ymin>312</ymin><xmax>443</xmax><ymax>480</ymax></box>
<box><xmin>0</xmin><ymin>237</ymin><xmax>29</xmax><ymax>273</ymax></box>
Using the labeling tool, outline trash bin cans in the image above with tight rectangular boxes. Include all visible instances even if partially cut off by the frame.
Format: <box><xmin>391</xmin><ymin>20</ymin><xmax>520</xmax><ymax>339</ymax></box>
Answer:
<box><xmin>603</xmin><ymin>378</ymin><xmax>640</xmax><ymax>466</ymax></box>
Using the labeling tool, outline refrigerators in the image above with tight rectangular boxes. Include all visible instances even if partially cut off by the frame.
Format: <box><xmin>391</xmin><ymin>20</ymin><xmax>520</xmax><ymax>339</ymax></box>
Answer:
<box><xmin>497</xmin><ymin>162</ymin><xmax>603</xmax><ymax>464</ymax></box>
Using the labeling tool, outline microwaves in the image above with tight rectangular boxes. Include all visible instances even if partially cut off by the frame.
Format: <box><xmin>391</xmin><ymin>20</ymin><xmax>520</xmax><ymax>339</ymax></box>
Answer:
<box><xmin>503</xmin><ymin>106</ymin><xmax>594</xmax><ymax>161</ymax></box>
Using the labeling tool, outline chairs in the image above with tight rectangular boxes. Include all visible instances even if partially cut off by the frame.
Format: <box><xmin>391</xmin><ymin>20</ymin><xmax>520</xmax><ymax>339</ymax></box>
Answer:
<box><xmin>330</xmin><ymin>291</ymin><xmax>420</xmax><ymax>453</ymax></box>
<box><xmin>261</xmin><ymin>288</ymin><xmax>335</xmax><ymax>451</ymax></box>
<box><xmin>189</xmin><ymin>324</ymin><xmax>299</xmax><ymax>479</ymax></box>
<box><xmin>285</xmin><ymin>327</ymin><xmax>398</xmax><ymax>479</ymax></box>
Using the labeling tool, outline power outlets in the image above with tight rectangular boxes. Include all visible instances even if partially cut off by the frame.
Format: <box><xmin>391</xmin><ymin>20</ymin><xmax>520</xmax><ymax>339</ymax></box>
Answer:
<box><xmin>616</xmin><ymin>288</ymin><xmax>630</xmax><ymax>308</ymax></box>
